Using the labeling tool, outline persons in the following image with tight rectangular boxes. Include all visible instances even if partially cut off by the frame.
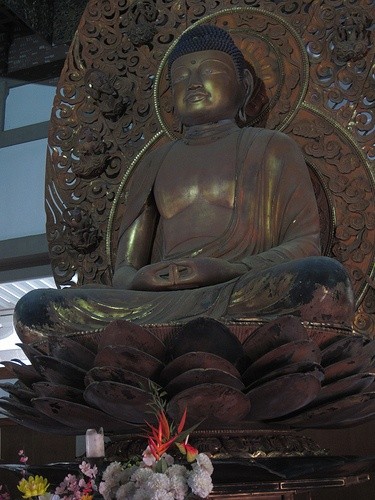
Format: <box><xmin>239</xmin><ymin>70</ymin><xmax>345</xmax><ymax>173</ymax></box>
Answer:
<box><xmin>12</xmin><ymin>25</ymin><xmax>354</xmax><ymax>333</ymax></box>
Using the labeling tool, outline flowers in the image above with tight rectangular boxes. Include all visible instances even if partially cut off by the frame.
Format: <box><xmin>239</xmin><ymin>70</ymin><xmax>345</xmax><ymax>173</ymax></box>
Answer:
<box><xmin>0</xmin><ymin>375</ymin><xmax>213</xmax><ymax>499</ymax></box>
